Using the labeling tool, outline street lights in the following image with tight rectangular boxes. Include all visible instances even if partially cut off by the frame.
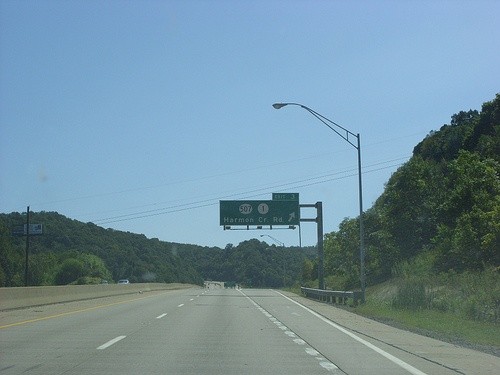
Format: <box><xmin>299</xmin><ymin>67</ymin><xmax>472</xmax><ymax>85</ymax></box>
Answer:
<box><xmin>271</xmin><ymin>101</ymin><xmax>366</xmax><ymax>304</ymax></box>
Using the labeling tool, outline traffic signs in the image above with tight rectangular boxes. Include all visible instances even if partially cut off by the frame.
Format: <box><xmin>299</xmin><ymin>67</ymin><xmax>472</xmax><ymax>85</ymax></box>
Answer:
<box><xmin>218</xmin><ymin>192</ymin><xmax>302</xmax><ymax>228</ymax></box>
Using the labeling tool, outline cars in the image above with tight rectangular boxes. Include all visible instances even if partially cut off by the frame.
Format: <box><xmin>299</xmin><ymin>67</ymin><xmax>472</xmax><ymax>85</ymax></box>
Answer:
<box><xmin>205</xmin><ymin>281</ymin><xmax>241</xmax><ymax>290</ymax></box>
<box><xmin>117</xmin><ymin>279</ymin><xmax>131</xmax><ymax>285</ymax></box>
